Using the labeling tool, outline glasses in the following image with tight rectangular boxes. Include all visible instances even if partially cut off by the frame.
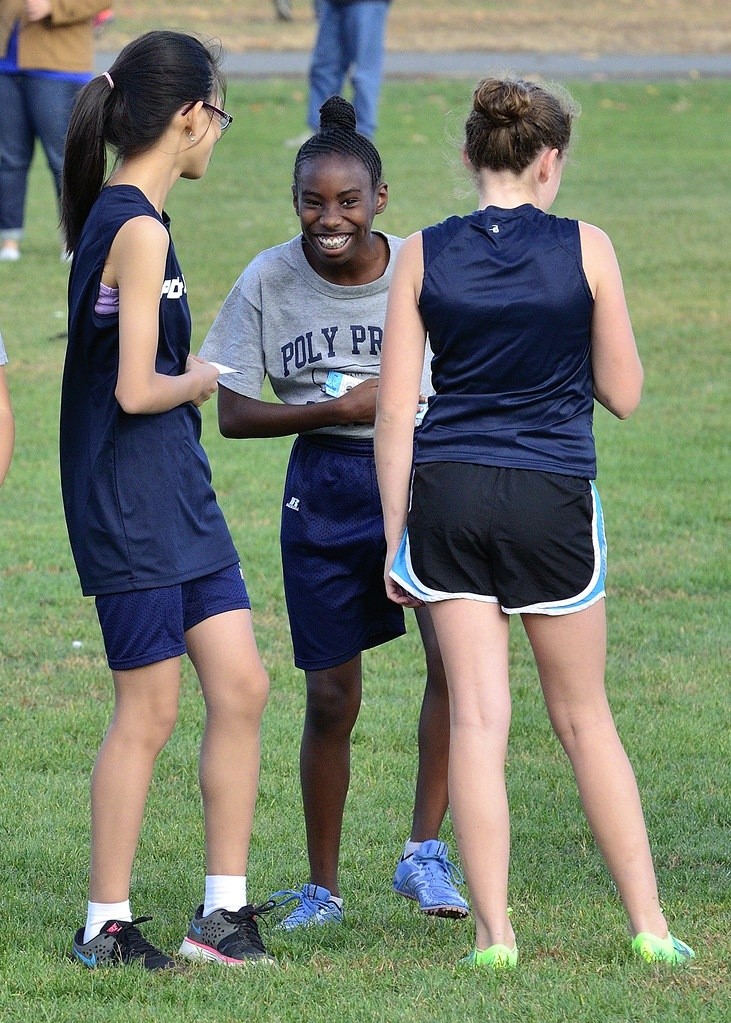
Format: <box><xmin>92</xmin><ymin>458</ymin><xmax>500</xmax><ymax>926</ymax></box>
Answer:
<box><xmin>181</xmin><ymin>98</ymin><xmax>234</xmax><ymax>131</ymax></box>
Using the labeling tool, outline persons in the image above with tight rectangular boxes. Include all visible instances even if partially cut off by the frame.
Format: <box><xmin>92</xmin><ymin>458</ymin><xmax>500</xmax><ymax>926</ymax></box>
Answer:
<box><xmin>373</xmin><ymin>71</ymin><xmax>695</xmax><ymax>975</ymax></box>
<box><xmin>58</xmin><ymin>29</ymin><xmax>299</xmax><ymax>973</ymax></box>
<box><xmin>196</xmin><ymin>97</ymin><xmax>470</xmax><ymax>938</ymax></box>
<box><xmin>0</xmin><ymin>330</ymin><xmax>22</xmax><ymax>491</ymax></box>
<box><xmin>0</xmin><ymin>0</ymin><xmax>117</xmax><ymax>260</ymax></box>
<box><xmin>273</xmin><ymin>0</ymin><xmax>392</xmax><ymax>147</ymax></box>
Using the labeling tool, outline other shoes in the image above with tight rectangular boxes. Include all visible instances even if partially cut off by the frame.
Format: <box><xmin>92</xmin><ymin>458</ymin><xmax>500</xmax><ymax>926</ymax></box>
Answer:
<box><xmin>2</xmin><ymin>245</ymin><xmax>18</xmax><ymax>262</ymax></box>
<box><xmin>60</xmin><ymin>247</ymin><xmax>74</xmax><ymax>262</ymax></box>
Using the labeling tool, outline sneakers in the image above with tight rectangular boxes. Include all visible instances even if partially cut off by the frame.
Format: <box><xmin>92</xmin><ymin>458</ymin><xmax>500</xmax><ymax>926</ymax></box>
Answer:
<box><xmin>389</xmin><ymin>840</ymin><xmax>468</xmax><ymax>920</ymax></box>
<box><xmin>632</xmin><ymin>932</ymin><xmax>695</xmax><ymax>964</ymax></box>
<box><xmin>178</xmin><ymin>899</ymin><xmax>275</xmax><ymax>970</ymax></box>
<box><xmin>460</xmin><ymin>907</ymin><xmax>515</xmax><ymax>966</ymax></box>
<box><xmin>72</xmin><ymin>915</ymin><xmax>183</xmax><ymax>974</ymax></box>
<box><xmin>269</xmin><ymin>884</ymin><xmax>343</xmax><ymax>934</ymax></box>
